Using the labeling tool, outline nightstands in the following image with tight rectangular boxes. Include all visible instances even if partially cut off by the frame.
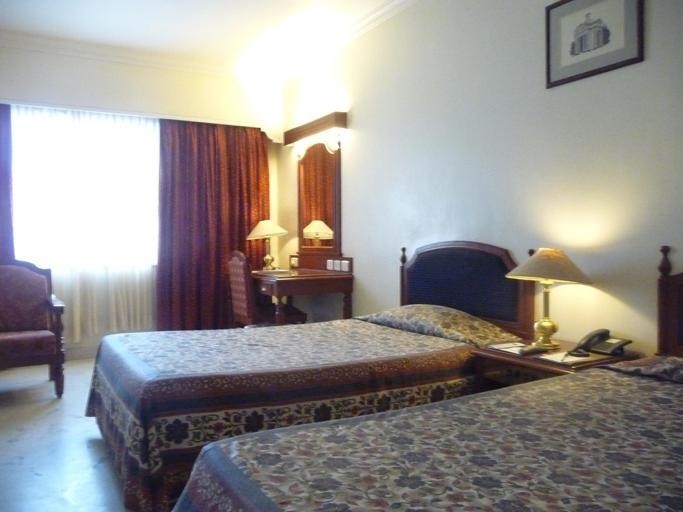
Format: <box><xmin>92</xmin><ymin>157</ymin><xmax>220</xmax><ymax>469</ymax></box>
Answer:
<box><xmin>470</xmin><ymin>336</ymin><xmax>644</xmax><ymax>395</ymax></box>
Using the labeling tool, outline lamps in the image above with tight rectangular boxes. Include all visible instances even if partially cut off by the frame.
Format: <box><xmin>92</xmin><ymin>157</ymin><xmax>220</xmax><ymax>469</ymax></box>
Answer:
<box><xmin>245</xmin><ymin>219</ymin><xmax>288</xmax><ymax>271</ymax></box>
<box><xmin>303</xmin><ymin>219</ymin><xmax>334</xmax><ymax>246</ymax></box>
<box><xmin>505</xmin><ymin>247</ymin><xmax>588</xmax><ymax>351</ymax></box>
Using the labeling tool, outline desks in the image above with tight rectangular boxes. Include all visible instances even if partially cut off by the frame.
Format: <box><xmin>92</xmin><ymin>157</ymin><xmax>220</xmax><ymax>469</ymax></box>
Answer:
<box><xmin>251</xmin><ymin>267</ymin><xmax>354</xmax><ymax>324</ymax></box>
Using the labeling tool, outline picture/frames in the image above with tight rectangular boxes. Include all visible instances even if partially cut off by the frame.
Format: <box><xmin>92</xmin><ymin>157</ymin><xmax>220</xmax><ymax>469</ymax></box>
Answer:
<box><xmin>545</xmin><ymin>0</ymin><xmax>645</xmax><ymax>90</ymax></box>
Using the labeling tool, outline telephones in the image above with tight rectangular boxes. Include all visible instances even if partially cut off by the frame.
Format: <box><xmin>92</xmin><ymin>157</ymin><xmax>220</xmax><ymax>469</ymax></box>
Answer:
<box><xmin>577</xmin><ymin>328</ymin><xmax>632</xmax><ymax>355</ymax></box>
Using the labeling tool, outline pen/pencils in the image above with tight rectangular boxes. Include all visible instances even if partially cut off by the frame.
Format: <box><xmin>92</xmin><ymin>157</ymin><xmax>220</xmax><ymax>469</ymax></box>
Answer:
<box><xmin>561</xmin><ymin>353</ymin><xmax>568</xmax><ymax>362</ymax></box>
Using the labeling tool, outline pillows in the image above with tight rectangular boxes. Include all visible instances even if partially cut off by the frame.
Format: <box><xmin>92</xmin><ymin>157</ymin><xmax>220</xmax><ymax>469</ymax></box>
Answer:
<box><xmin>358</xmin><ymin>303</ymin><xmax>523</xmax><ymax>344</ymax></box>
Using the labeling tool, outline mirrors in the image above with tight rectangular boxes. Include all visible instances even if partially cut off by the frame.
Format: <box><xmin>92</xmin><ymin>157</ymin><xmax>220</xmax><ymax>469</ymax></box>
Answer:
<box><xmin>297</xmin><ymin>142</ymin><xmax>342</xmax><ymax>256</ymax></box>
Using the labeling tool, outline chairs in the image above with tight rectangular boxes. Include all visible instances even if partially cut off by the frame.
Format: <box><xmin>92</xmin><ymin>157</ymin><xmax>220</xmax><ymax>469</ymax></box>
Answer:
<box><xmin>0</xmin><ymin>260</ymin><xmax>66</xmax><ymax>399</ymax></box>
<box><xmin>227</xmin><ymin>250</ymin><xmax>307</xmax><ymax>328</ymax></box>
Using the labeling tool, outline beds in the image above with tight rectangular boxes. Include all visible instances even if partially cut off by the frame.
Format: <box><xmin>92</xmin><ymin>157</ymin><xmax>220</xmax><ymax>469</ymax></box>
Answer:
<box><xmin>170</xmin><ymin>244</ymin><xmax>683</xmax><ymax>512</ymax></box>
<box><xmin>84</xmin><ymin>239</ymin><xmax>537</xmax><ymax>512</ymax></box>
